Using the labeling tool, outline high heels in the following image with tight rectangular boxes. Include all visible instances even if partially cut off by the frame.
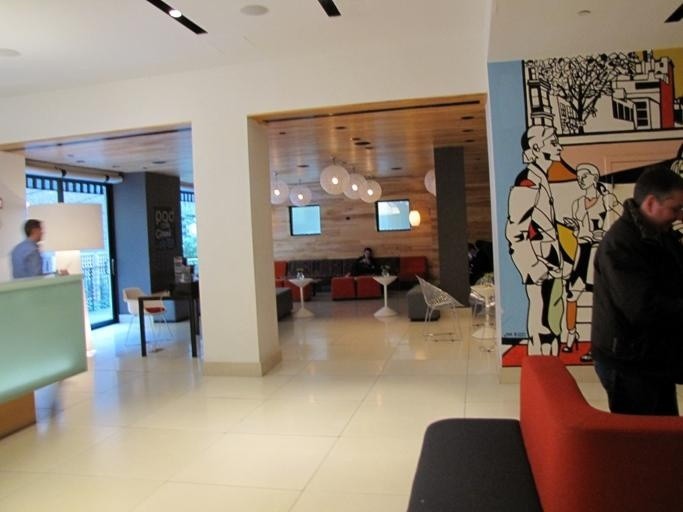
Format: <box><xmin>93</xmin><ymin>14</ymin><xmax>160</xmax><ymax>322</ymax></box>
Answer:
<box><xmin>562</xmin><ymin>331</ymin><xmax>581</xmax><ymax>353</ymax></box>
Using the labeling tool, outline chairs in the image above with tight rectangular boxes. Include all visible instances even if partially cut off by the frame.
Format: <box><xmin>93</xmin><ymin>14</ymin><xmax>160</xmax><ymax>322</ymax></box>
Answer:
<box><xmin>416</xmin><ymin>275</ymin><xmax>465</xmax><ymax>343</ymax></box>
<box><xmin>469</xmin><ymin>273</ymin><xmax>495</xmax><ymax>327</ymax></box>
<box><xmin>122</xmin><ymin>287</ymin><xmax>173</xmax><ymax>348</ymax></box>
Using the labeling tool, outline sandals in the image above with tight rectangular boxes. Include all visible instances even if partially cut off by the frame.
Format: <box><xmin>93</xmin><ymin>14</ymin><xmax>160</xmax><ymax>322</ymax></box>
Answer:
<box><xmin>580</xmin><ymin>350</ymin><xmax>592</xmax><ymax>362</ymax></box>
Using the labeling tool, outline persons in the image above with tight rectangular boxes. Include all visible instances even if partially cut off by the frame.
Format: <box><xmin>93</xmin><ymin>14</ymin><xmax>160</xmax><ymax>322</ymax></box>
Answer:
<box><xmin>590</xmin><ymin>166</ymin><xmax>683</xmax><ymax>416</ymax></box>
<box><xmin>466</xmin><ymin>235</ymin><xmax>494</xmax><ymax>289</ymax></box>
<box><xmin>344</xmin><ymin>247</ymin><xmax>374</xmax><ymax>277</ymax></box>
<box><xmin>12</xmin><ymin>218</ymin><xmax>69</xmax><ymax>279</ymax></box>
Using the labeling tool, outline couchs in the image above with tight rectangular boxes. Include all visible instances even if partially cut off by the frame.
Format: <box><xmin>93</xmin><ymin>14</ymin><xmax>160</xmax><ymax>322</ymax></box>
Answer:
<box><xmin>274</xmin><ymin>256</ymin><xmax>427</xmax><ymax>319</ymax></box>
<box><xmin>405</xmin><ymin>355</ymin><xmax>683</xmax><ymax>512</ymax></box>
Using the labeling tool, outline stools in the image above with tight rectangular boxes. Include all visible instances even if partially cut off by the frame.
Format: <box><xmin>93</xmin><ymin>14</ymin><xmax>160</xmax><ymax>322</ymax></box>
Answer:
<box><xmin>408</xmin><ymin>284</ymin><xmax>440</xmax><ymax>321</ymax></box>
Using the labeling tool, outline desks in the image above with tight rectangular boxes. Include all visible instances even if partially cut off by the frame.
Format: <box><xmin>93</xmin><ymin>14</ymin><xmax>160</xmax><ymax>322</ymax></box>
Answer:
<box><xmin>138</xmin><ymin>283</ymin><xmax>200</xmax><ymax>357</ymax></box>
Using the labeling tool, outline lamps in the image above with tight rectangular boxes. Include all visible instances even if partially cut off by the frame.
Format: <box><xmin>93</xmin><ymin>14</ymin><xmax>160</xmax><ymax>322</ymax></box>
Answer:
<box><xmin>270</xmin><ymin>156</ymin><xmax>437</xmax><ymax>207</ymax></box>
